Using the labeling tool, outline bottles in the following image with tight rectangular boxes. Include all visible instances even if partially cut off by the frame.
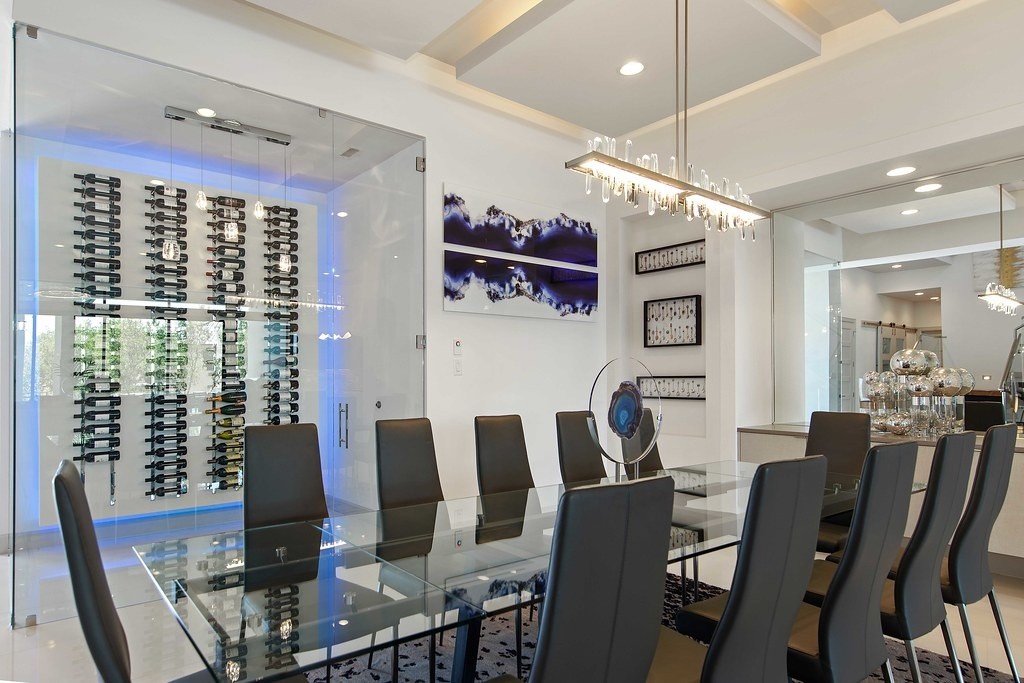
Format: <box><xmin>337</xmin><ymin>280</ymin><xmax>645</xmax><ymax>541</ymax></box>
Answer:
<box><xmin>262</xmin><ymin>205</ymin><xmax>301</xmax><ymax>426</ymax></box>
<box><xmin>143</xmin><ymin>182</ymin><xmax>189</xmax><ymax>497</ymax></box>
<box><xmin>72</xmin><ymin>171</ymin><xmax>121</xmax><ymax>463</ymax></box>
<box><xmin>204</xmin><ymin>192</ymin><xmax>247</xmax><ymax>492</ymax></box>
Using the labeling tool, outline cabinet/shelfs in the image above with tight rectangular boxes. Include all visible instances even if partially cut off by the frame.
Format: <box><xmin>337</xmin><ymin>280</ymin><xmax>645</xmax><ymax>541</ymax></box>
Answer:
<box><xmin>82</xmin><ymin>176</ymin><xmax>115</xmax><ymax>506</ymax></box>
<box><xmin>267</xmin><ymin>585</ymin><xmax>293</xmax><ymax>669</ymax></box>
<box><xmin>211</xmin><ymin>202</ymin><xmax>241</xmax><ymax>490</ymax></box>
<box><xmin>267</xmin><ymin>208</ymin><xmax>292</xmax><ymax>426</ymax></box>
<box><xmin>151</xmin><ymin>190</ymin><xmax>183</xmax><ymax>498</ymax></box>
<box><xmin>214</xmin><ymin>532</ymin><xmax>242</xmax><ymax>683</ymax></box>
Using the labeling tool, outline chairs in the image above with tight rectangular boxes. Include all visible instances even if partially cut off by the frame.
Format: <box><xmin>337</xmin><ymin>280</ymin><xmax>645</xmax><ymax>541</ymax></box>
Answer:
<box><xmin>53</xmin><ymin>458</ymin><xmax>307</xmax><ymax>683</ymax></box>
<box><xmin>647</xmin><ymin>455</ymin><xmax>827</xmax><ymax>683</ymax></box>
<box><xmin>687</xmin><ymin>439</ymin><xmax>919</xmax><ymax>683</ymax></box>
<box><xmin>375</xmin><ymin>417</ymin><xmax>524</xmax><ymax>683</ymax></box>
<box><xmin>803</xmin><ymin>432</ymin><xmax>976</xmax><ymax>683</ymax></box>
<box><xmin>529</xmin><ymin>475</ymin><xmax>674</xmax><ymax>683</ymax></box>
<box><xmin>619</xmin><ymin>408</ymin><xmax>740</xmax><ymax>607</ymax></box>
<box><xmin>557</xmin><ymin>410</ymin><xmax>700</xmax><ymax>605</ymax></box>
<box><xmin>825</xmin><ymin>422</ymin><xmax>1019</xmax><ymax>683</ymax></box>
<box><xmin>475</xmin><ymin>414</ymin><xmax>553</xmax><ymax>683</ymax></box>
<box><xmin>238</xmin><ymin>423</ymin><xmax>400</xmax><ymax>683</ymax></box>
<box><xmin>964</xmin><ymin>393</ymin><xmax>1007</xmax><ymax>432</ymax></box>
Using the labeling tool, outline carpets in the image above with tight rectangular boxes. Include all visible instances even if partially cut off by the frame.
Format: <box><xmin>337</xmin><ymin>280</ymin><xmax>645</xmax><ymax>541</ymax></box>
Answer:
<box><xmin>303</xmin><ymin>573</ymin><xmax>1024</xmax><ymax>683</ymax></box>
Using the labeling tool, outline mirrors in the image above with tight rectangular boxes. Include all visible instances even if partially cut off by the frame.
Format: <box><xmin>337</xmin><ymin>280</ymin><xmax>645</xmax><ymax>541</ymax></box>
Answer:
<box><xmin>771</xmin><ymin>160</ymin><xmax>1024</xmax><ymax>435</ymax></box>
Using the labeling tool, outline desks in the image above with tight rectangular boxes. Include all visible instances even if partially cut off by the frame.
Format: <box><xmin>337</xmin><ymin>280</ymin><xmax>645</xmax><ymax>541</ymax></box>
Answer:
<box><xmin>307</xmin><ymin>460</ymin><xmax>927</xmax><ymax>683</ymax></box>
<box><xmin>131</xmin><ymin>522</ymin><xmax>486</xmax><ymax>683</ymax></box>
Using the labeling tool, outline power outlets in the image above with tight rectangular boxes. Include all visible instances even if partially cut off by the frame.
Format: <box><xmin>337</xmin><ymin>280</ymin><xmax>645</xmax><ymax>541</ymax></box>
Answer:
<box><xmin>455</xmin><ymin>531</ymin><xmax>463</xmax><ymax>549</ymax></box>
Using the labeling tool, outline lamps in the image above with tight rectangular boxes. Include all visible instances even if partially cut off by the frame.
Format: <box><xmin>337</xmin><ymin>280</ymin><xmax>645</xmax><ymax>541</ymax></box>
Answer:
<box><xmin>564</xmin><ymin>0</ymin><xmax>772</xmax><ymax>243</ymax></box>
<box><xmin>978</xmin><ymin>184</ymin><xmax>1024</xmax><ymax>315</ymax></box>
<box><xmin>279</xmin><ymin>145</ymin><xmax>291</xmax><ymax>273</ymax></box>
<box><xmin>163</xmin><ymin>116</ymin><xmax>180</xmax><ymax>260</ymax></box>
<box><xmin>224</xmin><ymin>132</ymin><xmax>238</xmax><ymax>242</ymax></box>
<box><xmin>196</xmin><ymin>124</ymin><xmax>207</xmax><ymax>211</ymax></box>
<box><xmin>254</xmin><ymin>139</ymin><xmax>264</xmax><ymax>220</ymax></box>
